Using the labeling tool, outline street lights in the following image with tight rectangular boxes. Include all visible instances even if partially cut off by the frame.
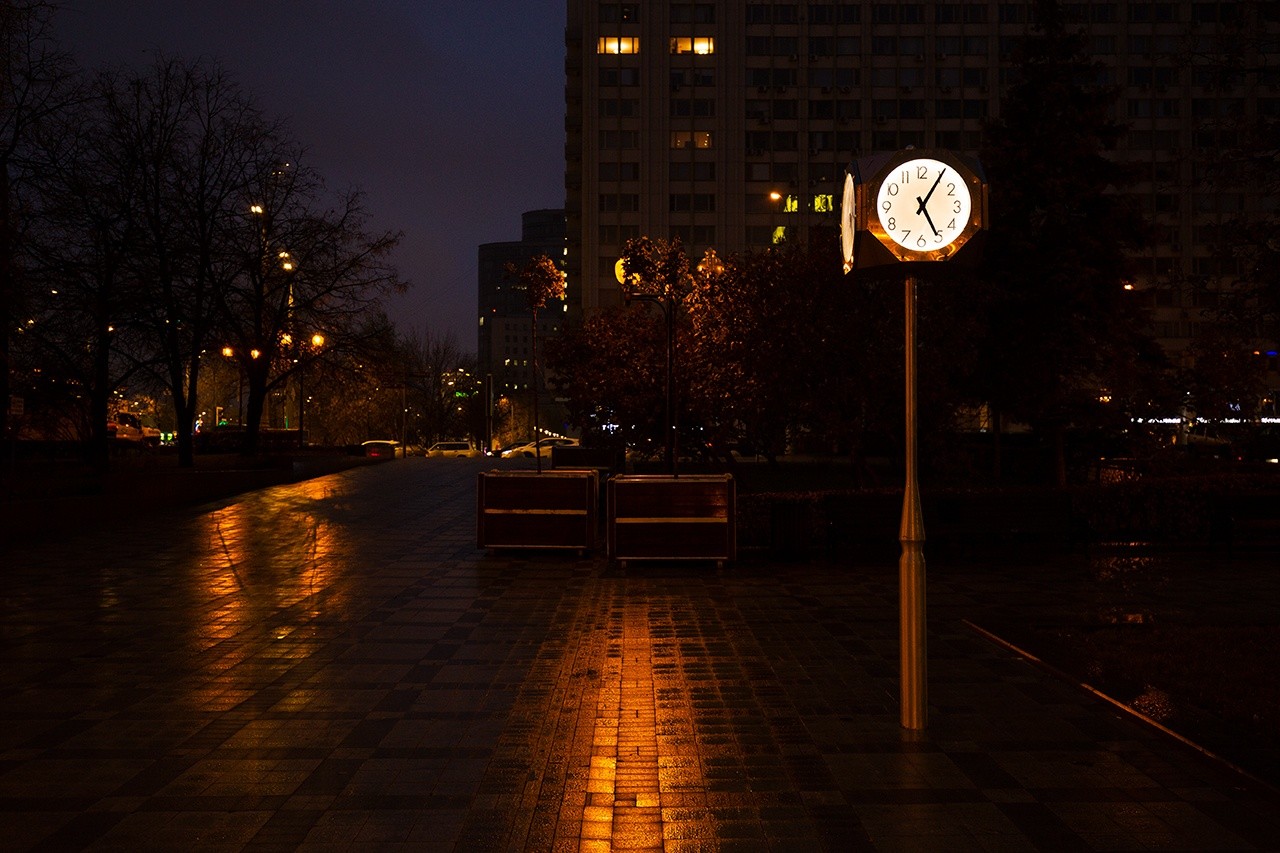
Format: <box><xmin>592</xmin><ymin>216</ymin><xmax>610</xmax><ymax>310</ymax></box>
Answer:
<box><xmin>631</xmin><ymin>291</ymin><xmax>672</xmax><ymax>462</ymax></box>
<box><xmin>281</xmin><ymin>332</ymin><xmax>325</xmax><ymax>448</ymax></box>
<box><xmin>222</xmin><ymin>347</ymin><xmax>260</xmax><ymax>432</ymax></box>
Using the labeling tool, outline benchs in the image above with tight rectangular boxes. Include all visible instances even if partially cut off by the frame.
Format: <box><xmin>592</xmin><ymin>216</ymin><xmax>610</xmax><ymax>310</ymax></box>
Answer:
<box><xmin>1212</xmin><ymin>491</ymin><xmax>1280</xmax><ymax>558</ymax></box>
<box><xmin>823</xmin><ymin>493</ymin><xmax>1082</xmax><ymax>568</ymax></box>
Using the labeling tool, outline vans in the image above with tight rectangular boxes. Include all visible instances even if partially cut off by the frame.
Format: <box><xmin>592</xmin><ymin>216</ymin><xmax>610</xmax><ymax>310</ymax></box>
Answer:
<box><xmin>424</xmin><ymin>440</ymin><xmax>477</xmax><ymax>459</ymax></box>
<box><xmin>103</xmin><ymin>409</ymin><xmax>180</xmax><ymax>449</ymax></box>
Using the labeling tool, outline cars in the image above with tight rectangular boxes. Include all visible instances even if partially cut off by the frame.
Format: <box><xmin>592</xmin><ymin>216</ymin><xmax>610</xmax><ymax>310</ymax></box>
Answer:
<box><xmin>476</xmin><ymin>436</ymin><xmax>579</xmax><ymax>459</ymax></box>
<box><xmin>275</xmin><ymin>439</ymin><xmax>429</xmax><ymax>484</ymax></box>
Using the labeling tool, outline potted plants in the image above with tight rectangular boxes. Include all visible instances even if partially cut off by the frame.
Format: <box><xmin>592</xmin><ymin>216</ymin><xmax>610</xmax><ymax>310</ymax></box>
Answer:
<box><xmin>605</xmin><ymin>234</ymin><xmax>737</xmax><ymax>562</ymax></box>
<box><xmin>476</xmin><ymin>252</ymin><xmax>597</xmax><ymax>554</ymax></box>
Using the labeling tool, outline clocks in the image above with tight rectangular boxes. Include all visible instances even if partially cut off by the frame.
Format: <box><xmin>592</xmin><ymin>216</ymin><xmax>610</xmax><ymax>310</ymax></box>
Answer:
<box><xmin>866</xmin><ymin>150</ymin><xmax>984</xmax><ymax>265</ymax></box>
